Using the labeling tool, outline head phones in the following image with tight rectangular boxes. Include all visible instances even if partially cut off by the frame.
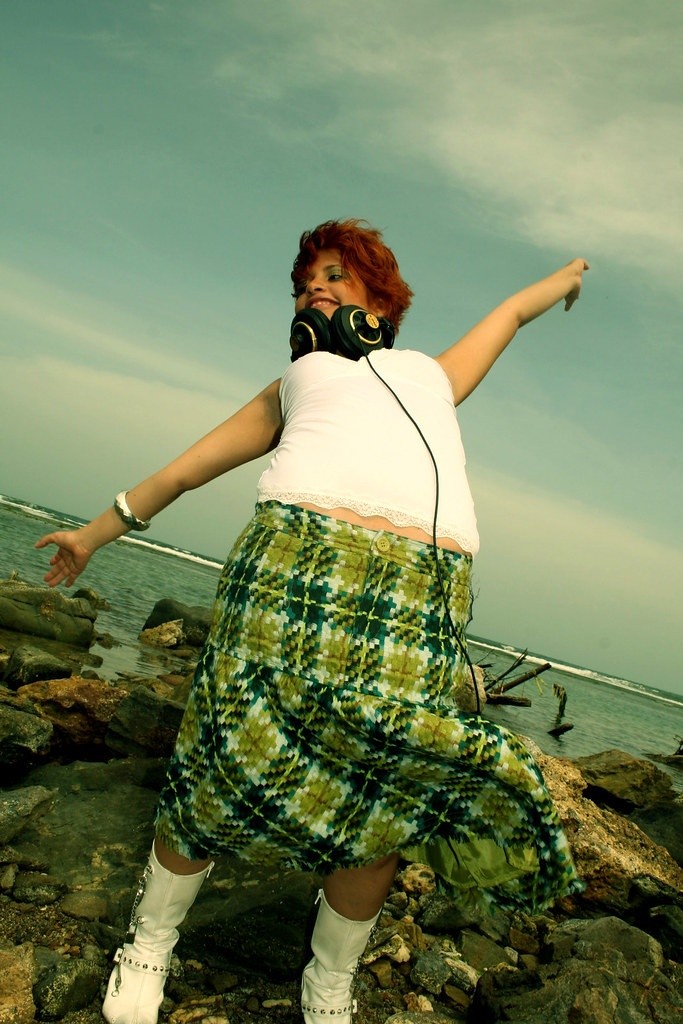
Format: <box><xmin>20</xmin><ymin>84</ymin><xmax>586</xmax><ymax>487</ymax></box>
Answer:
<box><xmin>289</xmin><ymin>305</ymin><xmax>396</xmax><ymax>362</ymax></box>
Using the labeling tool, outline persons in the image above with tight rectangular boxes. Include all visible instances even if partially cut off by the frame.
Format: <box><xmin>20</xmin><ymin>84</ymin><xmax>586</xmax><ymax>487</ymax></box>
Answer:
<box><xmin>34</xmin><ymin>218</ymin><xmax>591</xmax><ymax>1024</ymax></box>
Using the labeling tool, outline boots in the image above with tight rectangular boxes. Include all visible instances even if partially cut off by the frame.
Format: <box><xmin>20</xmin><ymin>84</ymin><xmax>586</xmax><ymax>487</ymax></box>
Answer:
<box><xmin>299</xmin><ymin>895</ymin><xmax>382</xmax><ymax>1024</ymax></box>
<box><xmin>102</xmin><ymin>837</ymin><xmax>215</xmax><ymax>1024</ymax></box>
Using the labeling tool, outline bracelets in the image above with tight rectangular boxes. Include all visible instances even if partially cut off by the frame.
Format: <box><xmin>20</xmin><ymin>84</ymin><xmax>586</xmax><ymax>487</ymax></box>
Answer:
<box><xmin>114</xmin><ymin>490</ymin><xmax>151</xmax><ymax>531</ymax></box>
